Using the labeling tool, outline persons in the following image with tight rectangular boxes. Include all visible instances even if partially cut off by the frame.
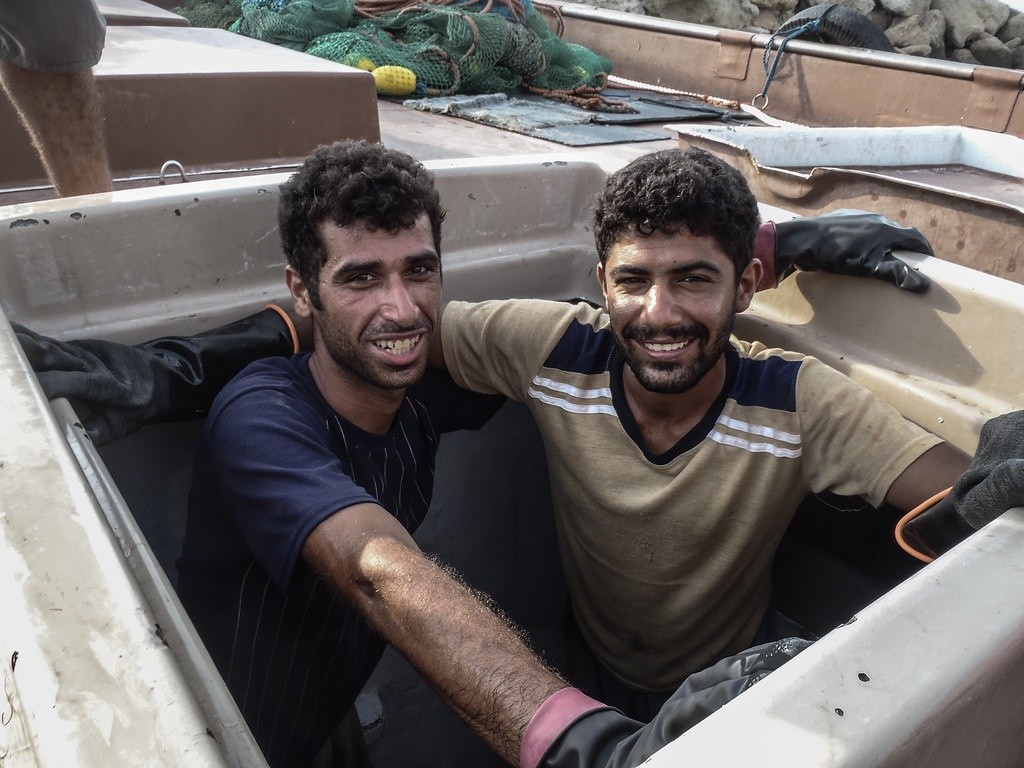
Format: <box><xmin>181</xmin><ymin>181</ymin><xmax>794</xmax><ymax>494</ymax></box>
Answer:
<box><xmin>177</xmin><ymin>139</ymin><xmax>935</xmax><ymax>767</ymax></box>
<box><xmin>6</xmin><ymin>148</ymin><xmax>1021</xmax><ymax>724</ymax></box>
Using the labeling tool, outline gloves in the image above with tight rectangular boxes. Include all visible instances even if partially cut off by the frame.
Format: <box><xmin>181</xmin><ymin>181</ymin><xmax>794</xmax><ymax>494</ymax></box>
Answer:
<box><xmin>895</xmin><ymin>410</ymin><xmax>1024</xmax><ymax>564</ymax></box>
<box><xmin>755</xmin><ymin>214</ymin><xmax>936</xmax><ymax>293</ymax></box>
<box><xmin>10</xmin><ymin>303</ymin><xmax>299</xmax><ymax>446</ymax></box>
<box><xmin>521</xmin><ymin>637</ymin><xmax>816</xmax><ymax>768</ymax></box>
